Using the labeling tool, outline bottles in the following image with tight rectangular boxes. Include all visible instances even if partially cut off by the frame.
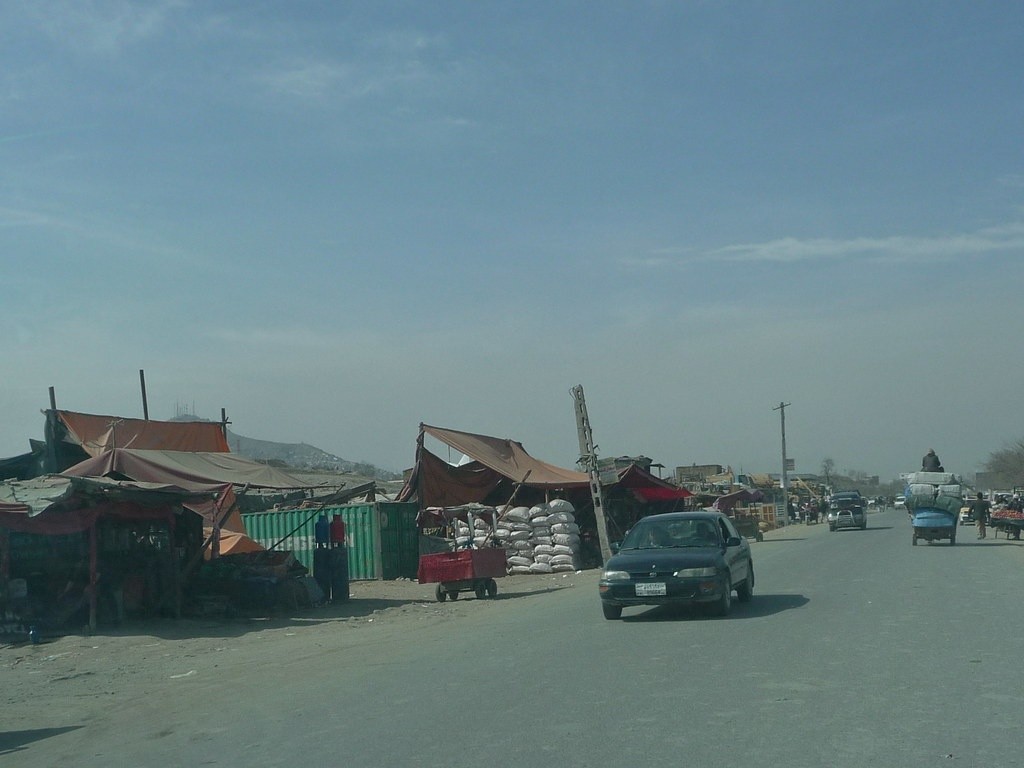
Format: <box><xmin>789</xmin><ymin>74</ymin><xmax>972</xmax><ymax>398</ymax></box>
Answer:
<box><xmin>329</xmin><ymin>514</ymin><xmax>344</xmax><ymax>541</ymax></box>
<box><xmin>315</xmin><ymin>516</ymin><xmax>328</xmax><ymax>541</ymax></box>
<box><xmin>29</xmin><ymin>626</ymin><xmax>39</xmax><ymax>644</ymax></box>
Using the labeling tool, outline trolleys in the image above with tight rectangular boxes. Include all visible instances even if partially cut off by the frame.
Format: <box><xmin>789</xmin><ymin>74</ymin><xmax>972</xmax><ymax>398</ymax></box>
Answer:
<box><xmin>989</xmin><ymin>517</ymin><xmax>1024</xmax><ymax>540</ymax></box>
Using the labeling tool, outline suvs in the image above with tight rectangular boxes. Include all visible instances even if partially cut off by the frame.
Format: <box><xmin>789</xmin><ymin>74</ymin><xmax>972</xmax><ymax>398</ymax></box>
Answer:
<box><xmin>827</xmin><ymin>492</ymin><xmax>867</xmax><ymax>531</ymax></box>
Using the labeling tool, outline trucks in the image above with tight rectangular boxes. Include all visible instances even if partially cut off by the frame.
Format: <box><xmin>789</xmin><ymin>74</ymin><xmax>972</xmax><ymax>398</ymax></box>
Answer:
<box><xmin>912</xmin><ymin>507</ymin><xmax>957</xmax><ymax>546</ymax></box>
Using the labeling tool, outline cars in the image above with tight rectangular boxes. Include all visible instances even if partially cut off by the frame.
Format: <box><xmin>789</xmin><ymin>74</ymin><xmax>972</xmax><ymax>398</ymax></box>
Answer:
<box><xmin>894</xmin><ymin>497</ymin><xmax>906</xmax><ymax>509</ymax></box>
<box><xmin>959</xmin><ymin>508</ymin><xmax>975</xmax><ymax>526</ymax></box>
<box><xmin>600</xmin><ymin>512</ymin><xmax>755</xmax><ymax>621</ymax></box>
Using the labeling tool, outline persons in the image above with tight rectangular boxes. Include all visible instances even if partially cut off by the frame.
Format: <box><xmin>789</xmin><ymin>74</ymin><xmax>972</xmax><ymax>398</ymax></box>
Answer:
<box><xmin>1007</xmin><ymin>494</ymin><xmax>1022</xmax><ymax>540</ymax></box>
<box><xmin>649</xmin><ymin>526</ymin><xmax>671</xmax><ymax>547</ymax></box>
<box><xmin>921</xmin><ymin>448</ymin><xmax>944</xmax><ymax>472</ymax></box>
<box><xmin>689</xmin><ymin>523</ymin><xmax>716</xmax><ymax>545</ymax></box>
<box><xmin>969</xmin><ymin>492</ymin><xmax>990</xmax><ymax>539</ymax></box>
